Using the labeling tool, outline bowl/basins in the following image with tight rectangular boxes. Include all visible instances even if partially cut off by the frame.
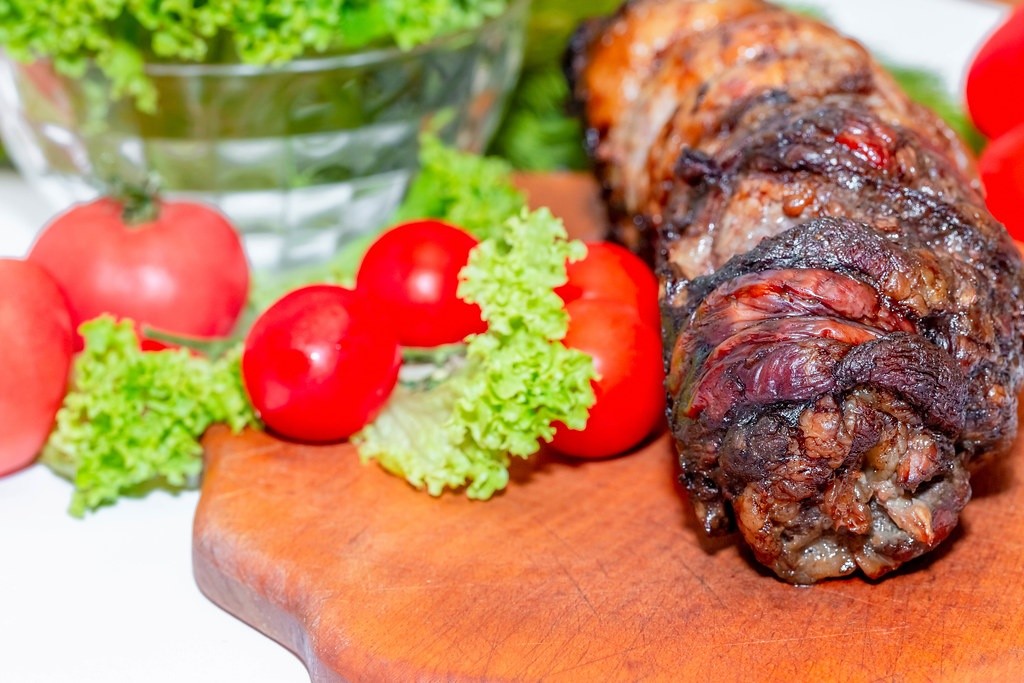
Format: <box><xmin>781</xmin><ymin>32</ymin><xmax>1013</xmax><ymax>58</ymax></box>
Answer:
<box><xmin>1</xmin><ymin>9</ymin><xmax>527</xmax><ymax>277</ymax></box>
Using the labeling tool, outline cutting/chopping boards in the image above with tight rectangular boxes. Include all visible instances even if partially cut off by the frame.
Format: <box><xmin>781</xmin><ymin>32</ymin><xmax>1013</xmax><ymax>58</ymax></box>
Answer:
<box><xmin>193</xmin><ymin>170</ymin><xmax>1024</xmax><ymax>683</ymax></box>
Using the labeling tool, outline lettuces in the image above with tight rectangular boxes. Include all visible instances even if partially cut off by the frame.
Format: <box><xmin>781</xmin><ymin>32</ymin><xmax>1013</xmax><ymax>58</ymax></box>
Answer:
<box><xmin>348</xmin><ymin>131</ymin><xmax>601</xmax><ymax>499</ymax></box>
<box><xmin>38</xmin><ymin>309</ymin><xmax>261</xmax><ymax>516</ymax></box>
<box><xmin>2</xmin><ymin>0</ymin><xmax>505</xmax><ymax>118</ymax></box>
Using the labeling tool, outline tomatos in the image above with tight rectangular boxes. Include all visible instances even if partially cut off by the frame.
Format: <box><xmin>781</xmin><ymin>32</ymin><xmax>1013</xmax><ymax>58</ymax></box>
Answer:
<box><xmin>238</xmin><ymin>284</ymin><xmax>400</xmax><ymax>446</ymax></box>
<box><xmin>538</xmin><ymin>241</ymin><xmax>666</xmax><ymax>461</ymax></box>
<box><xmin>27</xmin><ymin>175</ymin><xmax>252</xmax><ymax>355</ymax></box>
<box><xmin>353</xmin><ymin>217</ymin><xmax>489</xmax><ymax>349</ymax></box>
<box><xmin>0</xmin><ymin>254</ymin><xmax>68</xmax><ymax>477</ymax></box>
